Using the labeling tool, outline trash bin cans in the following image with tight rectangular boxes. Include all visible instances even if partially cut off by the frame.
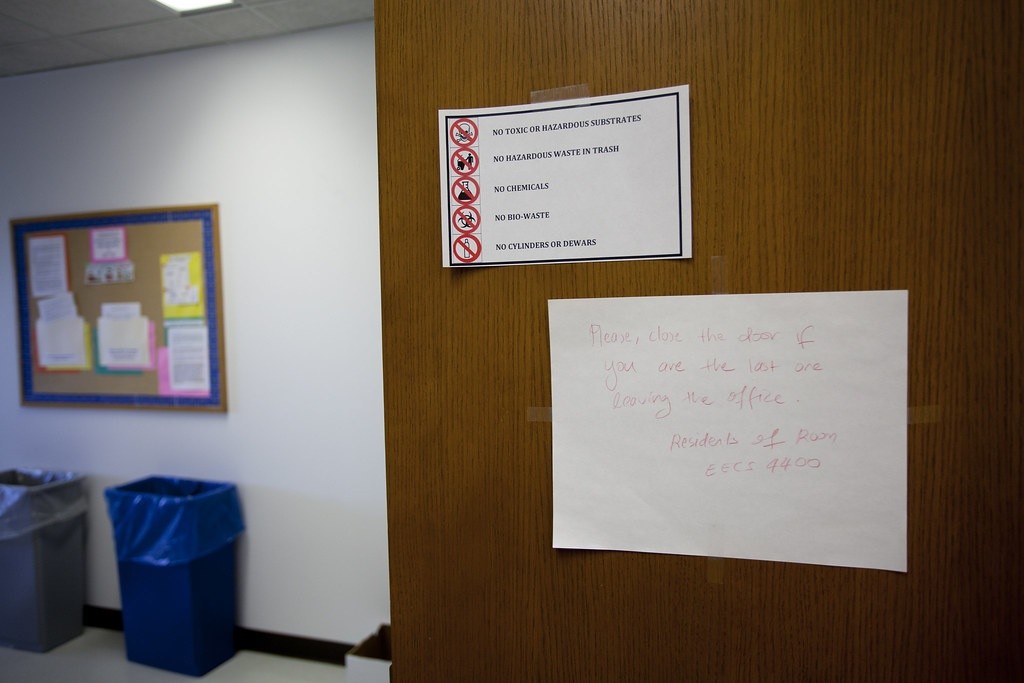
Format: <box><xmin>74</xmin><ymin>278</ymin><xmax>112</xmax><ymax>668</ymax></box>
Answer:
<box><xmin>104</xmin><ymin>474</ymin><xmax>240</xmax><ymax>677</ymax></box>
<box><xmin>0</xmin><ymin>467</ymin><xmax>90</xmax><ymax>652</ymax></box>
